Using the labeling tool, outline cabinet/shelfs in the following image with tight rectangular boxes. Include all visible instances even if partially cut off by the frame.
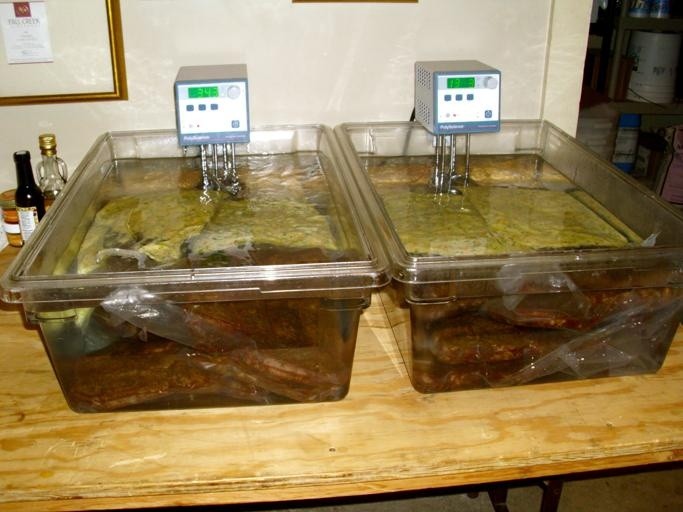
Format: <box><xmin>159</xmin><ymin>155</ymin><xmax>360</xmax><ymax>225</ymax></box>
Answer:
<box><xmin>574</xmin><ymin>1</ymin><xmax>683</xmax><ymax>189</ymax></box>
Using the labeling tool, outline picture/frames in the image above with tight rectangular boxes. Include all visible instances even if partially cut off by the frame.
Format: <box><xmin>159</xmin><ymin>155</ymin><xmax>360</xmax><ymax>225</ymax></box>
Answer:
<box><xmin>1</xmin><ymin>0</ymin><xmax>129</xmax><ymax>106</ymax></box>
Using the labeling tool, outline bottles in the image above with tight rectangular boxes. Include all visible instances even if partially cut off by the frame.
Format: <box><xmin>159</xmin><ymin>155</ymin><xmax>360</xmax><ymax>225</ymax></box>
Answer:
<box><xmin>13</xmin><ymin>133</ymin><xmax>67</xmax><ymax>245</ymax></box>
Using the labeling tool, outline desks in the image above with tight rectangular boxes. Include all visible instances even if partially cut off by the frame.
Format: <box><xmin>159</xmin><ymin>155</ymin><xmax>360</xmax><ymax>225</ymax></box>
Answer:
<box><xmin>0</xmin><ymin>292</ymin><xmax>683</xmax><ymax>510</ymax></box>
